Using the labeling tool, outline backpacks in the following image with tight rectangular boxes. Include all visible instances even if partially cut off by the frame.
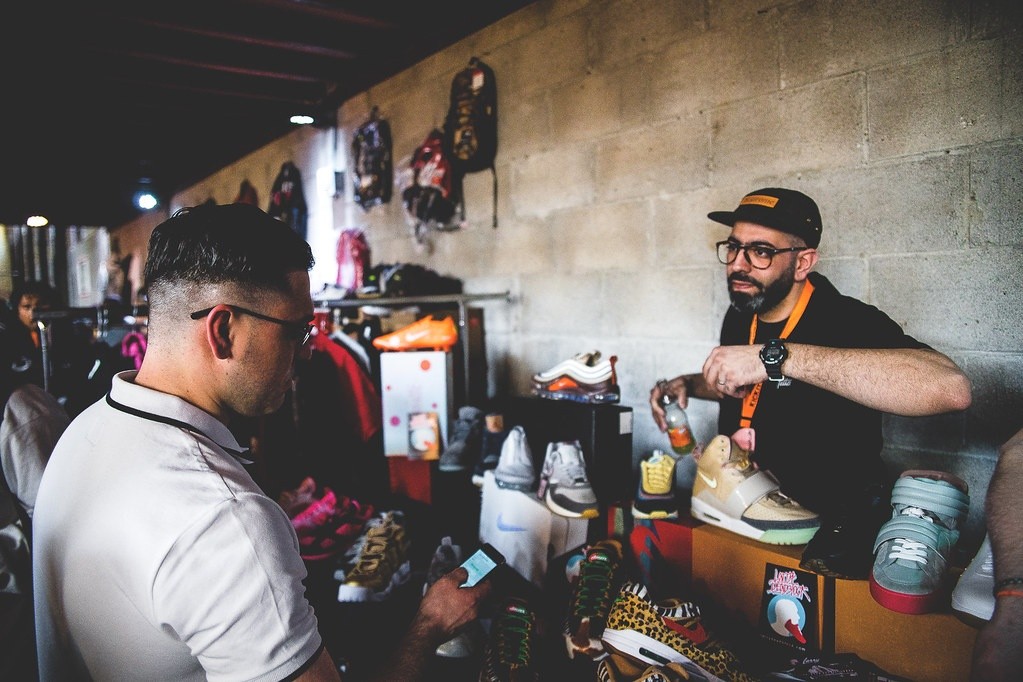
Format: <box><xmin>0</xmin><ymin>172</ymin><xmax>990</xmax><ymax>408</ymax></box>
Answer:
<box><xmin>349</xmin><ymin>105</ymin><xmax>397</xmax><ymax>210</ymax></box>
<box><xmin>328</xmin><ymin>231</ymin><xmax>372</xmax><ymax>294</ymax></box>
<box><xmin>234</xmin><ymin>180</ymin><xmax>258</xmax><ymax>207</ymax></box>
<box><xmin>443</xmin><ymin>59</ymin><xmax>500</xmax><ymax>174</ymax></box>
<box><xmin>400</xmin><ymin>129</ymin><xmax>466</xmax><ymax>228</ymax></box>
<box><xmin>264</xmin><ymin>162</ymin><xmax>307</xmax><ymax>238</ymax></box>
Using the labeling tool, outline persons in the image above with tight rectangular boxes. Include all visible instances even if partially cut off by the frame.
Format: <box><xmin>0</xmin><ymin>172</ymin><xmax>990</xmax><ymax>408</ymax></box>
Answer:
<box><xmin>0</xmin><ymin>280</ymin><xmax>73</xmax><ymax>395</ymax></box>
<box><xmin>650</xmin><ymin>187</ymin><xmax>973</xmax><ymax>539</ymax></box>
<box><xmin>975</xmin><ymin>429</ymin><xmax>1022</xmax><ymax>682</ymax></box>
<box><xmin>30</xmin><ymin>199</ymin><xmax>492</xmax><ymax>682</ymax></box>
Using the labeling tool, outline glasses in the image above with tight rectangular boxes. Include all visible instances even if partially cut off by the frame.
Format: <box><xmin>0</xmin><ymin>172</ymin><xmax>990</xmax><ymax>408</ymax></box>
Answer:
<box><xmin>188</xmin><ymin>300</ymin><xmax>317</xmax><ymax>347</ymax></box>
<box><xmin>716</xmin><ymin>240</ymin><xmax>808</xmax><ymax>271</ymax></box>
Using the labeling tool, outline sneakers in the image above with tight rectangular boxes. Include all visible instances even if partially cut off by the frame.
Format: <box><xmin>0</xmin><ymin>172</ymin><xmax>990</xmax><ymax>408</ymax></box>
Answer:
<box><xmin>269</xmin><ymin>312</ymin><xmax>968</xmax><ymax>682</ymax></box>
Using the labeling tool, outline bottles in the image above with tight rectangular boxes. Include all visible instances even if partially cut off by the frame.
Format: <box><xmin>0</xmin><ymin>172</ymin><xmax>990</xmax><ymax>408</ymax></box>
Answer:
<box><xmin>663</xmin><ymin>396</ymin><xmax>697</xmax><ymax>455</ymax></box>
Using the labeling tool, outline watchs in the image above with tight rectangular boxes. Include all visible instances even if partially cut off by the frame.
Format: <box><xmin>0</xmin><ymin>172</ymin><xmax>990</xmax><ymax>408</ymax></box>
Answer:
<box><xmin>759</xmin><ymin>337</ymin><xmax>793</xmax><ymax>387</ymax></box>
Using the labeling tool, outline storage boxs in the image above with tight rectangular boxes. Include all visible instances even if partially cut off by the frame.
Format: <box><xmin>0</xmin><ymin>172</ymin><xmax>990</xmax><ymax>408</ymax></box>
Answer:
<box><xmin>608</xmin><ymin>500</ymin><xmax>839</xmax><ymax>658</ymax></box>
<box><xmin>495</xmin><ymin>396</ymin><xmax>634</xmax><ymax>491</ymax></box>
<box><xmin>835</xmin><ymin>542</ymin><xmax>987</xmax><ymax>682</ymax></box>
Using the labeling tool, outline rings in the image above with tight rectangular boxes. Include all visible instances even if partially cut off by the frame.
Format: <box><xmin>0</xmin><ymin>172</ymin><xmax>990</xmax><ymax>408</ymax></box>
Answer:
<box><xmin>716</xmin><ymin>379</ymin><xmax>726</xmax><ymax>386</ymax></box>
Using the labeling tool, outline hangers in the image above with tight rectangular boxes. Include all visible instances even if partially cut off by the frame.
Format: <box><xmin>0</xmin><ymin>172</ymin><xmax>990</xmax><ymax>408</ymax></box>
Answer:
<box><xmin>92</xmin><ymin>296</ymin><xmax>374</xmax><ymax>343</ymax></box>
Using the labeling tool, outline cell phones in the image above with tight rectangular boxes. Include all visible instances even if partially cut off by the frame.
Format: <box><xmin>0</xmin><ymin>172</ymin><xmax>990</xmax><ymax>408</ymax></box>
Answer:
<box><xmin>458</xmin><ymin>543</ymin><xmax>506</xmax><ymax>589</ymax></box>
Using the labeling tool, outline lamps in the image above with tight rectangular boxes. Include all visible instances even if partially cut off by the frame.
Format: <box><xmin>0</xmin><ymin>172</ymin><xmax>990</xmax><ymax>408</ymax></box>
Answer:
<box><xmin>287</xmin><ymin>104</ymin><xmax>338</xmax><ymax>130</ymax></box>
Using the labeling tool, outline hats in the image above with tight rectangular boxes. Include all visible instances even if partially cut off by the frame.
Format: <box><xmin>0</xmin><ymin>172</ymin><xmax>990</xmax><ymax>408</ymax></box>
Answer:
<box><xmin>707</xmin><ymin>187</ymin><xmax>824</xmax><ymax>251</ymax></box>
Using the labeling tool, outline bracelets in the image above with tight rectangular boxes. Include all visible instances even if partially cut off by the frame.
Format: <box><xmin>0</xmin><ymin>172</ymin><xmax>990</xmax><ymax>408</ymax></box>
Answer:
<box><xmin>994</xmin><ymin>577</ymin><xmax>1023</xmax><ymax>602</ymax></box>
